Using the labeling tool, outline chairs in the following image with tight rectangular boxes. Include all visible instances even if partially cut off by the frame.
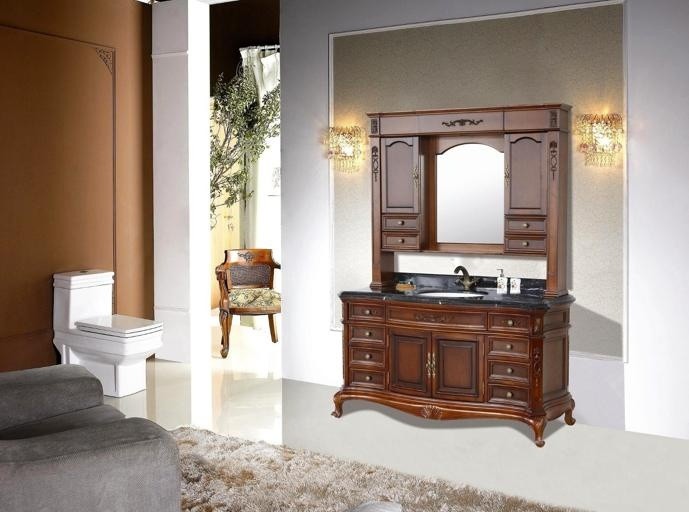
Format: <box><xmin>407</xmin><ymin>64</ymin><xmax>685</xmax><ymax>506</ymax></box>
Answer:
<box><xmin>215</xmin><ymin>249</ymin><xmax>281</xmax><ymax>359</ymax></box>
<box><xmin>0</xmin><ymin>364</ymin><xmax>181</xmax><ymax>512</ymax></box>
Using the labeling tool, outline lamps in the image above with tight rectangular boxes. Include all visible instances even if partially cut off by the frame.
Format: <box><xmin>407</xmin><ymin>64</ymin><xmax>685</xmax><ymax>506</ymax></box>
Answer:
<box><xmin>575</xmin><ymin>113</ymin><xmax>623</xmax><ymax>167</ymax></box>
<box><xmin>320</xmin><ymin>126</ymin><xmax>369</xmax><ymax>172</ymax></box>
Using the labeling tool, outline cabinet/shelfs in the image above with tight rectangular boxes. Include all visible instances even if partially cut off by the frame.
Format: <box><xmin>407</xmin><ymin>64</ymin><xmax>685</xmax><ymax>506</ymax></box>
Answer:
<box><xmin>385</xmin><ymin>300</ymin><xmax>487</xmax><ymax>420</ymax></box>
<box><xmin>366</xmin><ymin>111</ymin><xmax>428</xmax><ymax>292</ymax></box>
<box><xmin>486</xmin><ymin>300</ymin><xmax>576</xmax><ymax>448</ymax></box>
<box><xmin>503</xmin><ymin>103</ymin><xmax>574</xmax><ymax>298</ymax></box>
<box><xmin>332</xmin><ymin>300</ymin><xmax>389</xmax><ymax>418</ymax></box>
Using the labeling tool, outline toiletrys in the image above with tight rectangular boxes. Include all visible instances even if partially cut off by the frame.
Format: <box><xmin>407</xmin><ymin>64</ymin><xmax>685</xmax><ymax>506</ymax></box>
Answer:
<box><xmin>496</xmin><ymin>269</ymin><xmax>508</xmax><ymax>295</ymax></box>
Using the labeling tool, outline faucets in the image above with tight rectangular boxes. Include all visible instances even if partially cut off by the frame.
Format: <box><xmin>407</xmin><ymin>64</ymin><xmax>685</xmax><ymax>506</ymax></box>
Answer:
<box><xmin>455</xmin><ymin>266</ymin><xmax>470</xmax><ymax>282</ymax></box>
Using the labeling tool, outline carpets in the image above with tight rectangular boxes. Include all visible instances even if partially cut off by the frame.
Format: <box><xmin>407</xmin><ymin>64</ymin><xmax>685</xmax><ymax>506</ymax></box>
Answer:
<box><xmin>171</xmin><ymin>427</ymin><xmax>584</xmax><ymax>512</ymax></box>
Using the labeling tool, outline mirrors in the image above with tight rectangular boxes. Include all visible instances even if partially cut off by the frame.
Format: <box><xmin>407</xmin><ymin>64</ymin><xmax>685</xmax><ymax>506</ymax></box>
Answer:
<box><xmin>427</xmin><ymin>133</ymin><xmax>504</xmax><ymax>253</ymax></box>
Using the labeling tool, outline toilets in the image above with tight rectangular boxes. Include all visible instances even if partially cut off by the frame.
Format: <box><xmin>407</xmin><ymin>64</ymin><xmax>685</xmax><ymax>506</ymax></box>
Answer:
<box><xmin>52</xmin><ymin>269</ymin><xmax>162</xmax><ymax>397</ymax></box>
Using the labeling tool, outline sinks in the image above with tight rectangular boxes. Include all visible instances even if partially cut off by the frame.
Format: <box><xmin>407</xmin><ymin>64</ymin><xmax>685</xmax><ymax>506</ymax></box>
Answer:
<box><xmin>417</xmin><ymin>290</ymin><xmax>488</xmax><ymax>298</ymax></box>
